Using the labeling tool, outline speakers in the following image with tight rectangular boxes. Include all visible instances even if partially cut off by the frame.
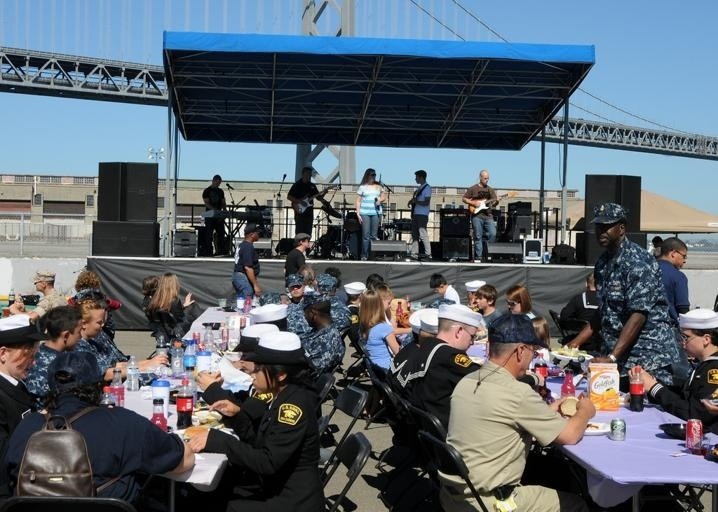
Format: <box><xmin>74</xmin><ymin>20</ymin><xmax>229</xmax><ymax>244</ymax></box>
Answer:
<box><xmin>234</xmin><ymin>237</ymin><xmax>272</xmax><ymax>257</ymax></box>
<box><xmin>585</xmin><ymin>174</ymin><xmax>641</xmax><ymax>233</ymax></box>
<box><xmin>576</xmin><ymin>232</ymin><xmax>648</xmax><ymax>267</ymax></box>
<box><xmin>370</xmin><ymin>240</ymin><xmax>407</xmax><ymax>261</ymax></box>
<box><xmin>97</xmin><ymin>161</ymin><xmax>158</xmax><ymax>222</ymax></box>
<box><xmin>482</xmin><ymin>240</ymin><xmax>523</xmax><ymax>262</ymax></box>
<box><xmin>92</xmin><ymin>221</ymin><xmax>160</xmax><ymax>257</ymax></box>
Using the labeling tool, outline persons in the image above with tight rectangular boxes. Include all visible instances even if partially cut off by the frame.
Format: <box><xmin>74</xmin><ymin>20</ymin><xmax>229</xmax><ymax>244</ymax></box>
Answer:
<box><xmin>286</xmin><ymin>166</ymin><xmax>330</xmax><ymax>259</ymax></box>
<box><xmin>201</xmin><ymin>174</ymin><xmax>228</xmax><ymax>256</ymax></box>
<box><xmin>407</xmin><ymin>169</ymin><xmax>434</xmax><ymax>261</ymax></box>
<box><xmin>462</xmin><ymin>169</ymin><xmax>500</xmax><ymax>264</ymax></box>
<box><xmin>355</xmin><ymin>167</ymin><xmax>386</xmax><ymax>261</ymax></box>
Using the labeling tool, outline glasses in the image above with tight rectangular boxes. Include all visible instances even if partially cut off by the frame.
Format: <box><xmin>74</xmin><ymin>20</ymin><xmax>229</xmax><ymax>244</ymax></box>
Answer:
<box><xmin>675</xmin><ymin>332</ymin><xmax>695</xmax><ymax>349</ymax></box>
<box><xmin>526</xmin><ymin>345</ymin><xmax>538</xmax><ymax>360</ymax></box>
<box><xmin>667</xmin><ymin>247</ymin><xmax>687</xmax><ymax>260</ymax></box>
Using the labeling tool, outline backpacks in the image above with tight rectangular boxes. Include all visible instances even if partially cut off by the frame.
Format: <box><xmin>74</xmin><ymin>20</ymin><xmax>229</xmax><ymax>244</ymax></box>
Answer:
<box><xmin>15</xmin><ymin>404</ymin><xmax>123</xmax><ymax>496</ymax></box>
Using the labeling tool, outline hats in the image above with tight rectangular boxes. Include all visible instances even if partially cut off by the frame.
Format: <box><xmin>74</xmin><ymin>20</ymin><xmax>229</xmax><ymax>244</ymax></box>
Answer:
<box><xmin>0</xmin><ymin>313</ymin><xmax>50</xmax><ymax>345</ymax></box>
<box><xmin>677</xmin><ymin>308</ymin><xmax>718</xmax><ymax>330</ymax></box>
<box><xmin>47</xmin><ymin>351</ymin><xmax>113</xmax><ymax>396</ymax></box>
<box><xmin>343</xmin><ymin>282</ymin><xmax>367</xmax><ymax>295</ymax></box>
<box><xmin>295</xmin><ymin>233</ymin><xmax>311</xmax><ymax>243</ymax></box>
<box><xmin>464</xmin><ymin>280</ymin><xmax>486</xmax><ymax>292</ymax></box>
<box><xmin>32</xmin><ymin>270</ymin><xmax>56</xmax><ymax>284</ymax></box>
<box><xmin>300</xmin><ymin>292</ymin><xmax>329</xmax><ymax>309</ymax></box>
<box><xmin>286</xmin><ymin>273</ymin><xmax>305</xmax><ymax>290</ymax></box>
<box><xmin>488</xmin><ymin>313</ymin><xmax>549</xmax><ymax>348</ymax></box>
<box><xmin>231</xmin><ymin>292</ymin><xmax>300</xmax><ymax>365</ymax></box>
<box><xmin>244</xmin><ymin>222</ymin><xmax>264</xmax><ymax>234</ymax></box>
<box><xmin>589</xmin><ymin>202</ymin><xmax>628</xmax><ymax>225</ymax></box>
<box><xmin>408</xmin><ymin>303</ymin><xmax>483</xmax><ymax>335</ymax></box>
<box><xmin>314</xmin><ymin>271</ymin><xmax>337</xmax><ymax>292</ymax></box>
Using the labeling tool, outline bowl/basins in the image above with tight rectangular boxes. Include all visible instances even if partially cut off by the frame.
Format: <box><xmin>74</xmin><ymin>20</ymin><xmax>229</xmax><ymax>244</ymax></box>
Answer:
<box><xmin>138</xmin><ymin>372</ymin><xmax>159</xmax><ymax>386</ymax></box>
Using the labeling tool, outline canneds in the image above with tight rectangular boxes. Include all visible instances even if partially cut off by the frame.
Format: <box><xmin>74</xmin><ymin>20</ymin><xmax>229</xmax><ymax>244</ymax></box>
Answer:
<box><xmin>222</xmin><ymin>329</ymin><xmax>229</xmax><ymax>342</ymax></box>
<box><xmin>685</xmin><ymin>418</ymin><xmax>704</xmax><ymax>450</ymax></box>
<box><xmin>192</xmin><ymin>332</ymin><xmax>200</xmax><ymax>344</ymax></box>
<box><xmin>240</xmin><ymin>317</ymin><xmax>246</xmax><ymax>328</ymax></box>
<box><xmin>611</xmin><ymin>419</ymin><xmax>626</xmax><ymax>441</ymax></box>
<box><xmin>198</xmin><ymin>343</ymin><xmax>206</xmax><ymax>352</ymax></box>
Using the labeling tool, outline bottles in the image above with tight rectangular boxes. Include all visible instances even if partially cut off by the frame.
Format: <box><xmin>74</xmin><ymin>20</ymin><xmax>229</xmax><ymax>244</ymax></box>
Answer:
<box><xmin>126</xmin><ymin>356</ymin><xmax>139</xmax><ymax>391</ymax></box>
<box><xmin>236</xmin><ymin>293</ymin><xmax>256</xmax><ymax>313</ymax></box>
<box><xmin>176</xmin><ymin>380</ymin><xmax>193</xmax><ymax>428</ymax></box>
<box><xmin>109</xmin><ymin>367</ymin><xmax>125</xmax><ymax>408</ymax></box>
<box><xmin>8</xmin><ymin>287</ymin><xmax>15</xmax><ymax>307</ymax></box>
<box><xmin>204</xmin><ymin>326</ymin><xmax>214</xmax><ymax>346</ymax></box>
<box><xmin>405</xmin><ymin>295</ymin><xmax>411</xmax><ymax>311</ymax></box>
<box><xmin>156</xmin><ymin>335</ymin><xmax>212</xmax><ymax>374</ymax></box>
<box><xmin>150</xmin><ymin>399</ymin><xmax>168</xmax><ymax>432</ymax></box>
<box><xmin>99</xmin><ymin>387</ymin><xmax>114</xmax><ymax>407</ymax></box>
<box><xmin>629</xmin><ymin>366</ymin><xmax>645</xmax><ymax>412</ymax></box>
<box><xmin>535</xmin><ymin>353</ymin><xmax>547</xmax><ymax>378</ymax></box>
<box><xmin>395</xmin><ymin>302</ymin><xmax>403</xmax><ymax>320</ymax></box>
<box><xmin>561</xmin><ymin>370</ymin><xmax>575</xmax><ymax>398</ymax></box>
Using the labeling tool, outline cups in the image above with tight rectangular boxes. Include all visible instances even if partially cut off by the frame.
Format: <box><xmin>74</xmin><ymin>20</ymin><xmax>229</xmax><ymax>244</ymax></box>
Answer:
<box><xmin>218</xmin><ymin>298</ymin><xmax>227</xmax><ymax>308</ymax></box>
<box><xmin>152</xmin><ymin>379</ymin><xmax>170</xmax><ymax>418</ymax></box>
<box><xmin>2</xmin><ymin>307</ymin><xmax>10</xmax><ymax>317</ymax></box>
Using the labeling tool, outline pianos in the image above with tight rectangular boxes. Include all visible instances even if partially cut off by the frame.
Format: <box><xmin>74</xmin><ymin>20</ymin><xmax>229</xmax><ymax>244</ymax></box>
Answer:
<box><xmin>201</xmin><ymin>210</ymin><xmax>263</xmax><ymax>220</ymax></box>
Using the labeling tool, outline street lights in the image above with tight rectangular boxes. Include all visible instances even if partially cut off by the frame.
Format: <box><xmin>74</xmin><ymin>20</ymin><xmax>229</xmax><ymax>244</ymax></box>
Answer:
<box><xmin>148</xmin><ymin>146</ymin><xmax>166</xmax><ymax>164</ymax></box>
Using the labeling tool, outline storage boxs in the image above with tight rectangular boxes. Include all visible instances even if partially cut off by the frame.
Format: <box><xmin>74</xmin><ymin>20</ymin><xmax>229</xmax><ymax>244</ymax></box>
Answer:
<box><xmin>170</xmin><ymin>229</ymin><xmax>198</xmax><ymax>258</ymax></box>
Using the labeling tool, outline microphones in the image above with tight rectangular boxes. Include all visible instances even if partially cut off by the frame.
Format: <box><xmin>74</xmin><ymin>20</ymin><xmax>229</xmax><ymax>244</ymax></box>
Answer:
<box><xmin>375</xmin><ymin>197</ymin><xmax>380</xmax><ymax>210</ymax></box>
<box><xmin>226</xmin><ymin>183</ymin><xmax>234</xmax><ymax>190</ymax></box>
<box><xmin>283</xmin><ymin>174</ymin><xmax>287</xmax><ymax>181</ymax></box>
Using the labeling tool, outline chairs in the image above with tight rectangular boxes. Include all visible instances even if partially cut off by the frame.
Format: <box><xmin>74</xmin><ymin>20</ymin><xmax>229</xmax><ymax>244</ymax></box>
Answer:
<box><xmin>318</xmin><ymin>310</ymin><xmax>716</xmax><ymax>512</ymax></box>
<box><xmin>0</xmin><ymin>493</ymin><xmax>134</xmax><ymax>512</ymax></box>
<box><xmin>146</xmin><ymin>308</ymin><xmax>179</xmax><ymax>360</ymax></box>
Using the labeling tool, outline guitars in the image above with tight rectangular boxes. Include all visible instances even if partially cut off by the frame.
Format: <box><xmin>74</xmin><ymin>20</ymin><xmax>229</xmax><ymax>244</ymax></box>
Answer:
<box><xmin>469</xmin><ymin>192</ymin><xmax>518</xmax><ymax>214</ymax></box>
<box><xmin>292</xmin><ymin>185</ymin><xmax>337</xmax><ymax>214</ymax></box>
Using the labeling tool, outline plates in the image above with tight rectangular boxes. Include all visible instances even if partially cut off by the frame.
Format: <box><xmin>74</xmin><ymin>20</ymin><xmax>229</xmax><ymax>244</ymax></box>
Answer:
<box><xmin>659</xmin><ymin>422</ymin><xmax>712</xmax><ymax>439</ymax></box>
<box><xmin>582</xmin><ymin>422</ymin><xmax>611</xmax><ymax>436</ymax></box>
<box><xmin>550</xmin><ymin>350</ymin><xmax>593</xmax><ymax>362</ymax></box>
<box><xmin>201</xmin><ymin>322</ymin><xmax>221</xmax><ymax>330</ymax></box>
